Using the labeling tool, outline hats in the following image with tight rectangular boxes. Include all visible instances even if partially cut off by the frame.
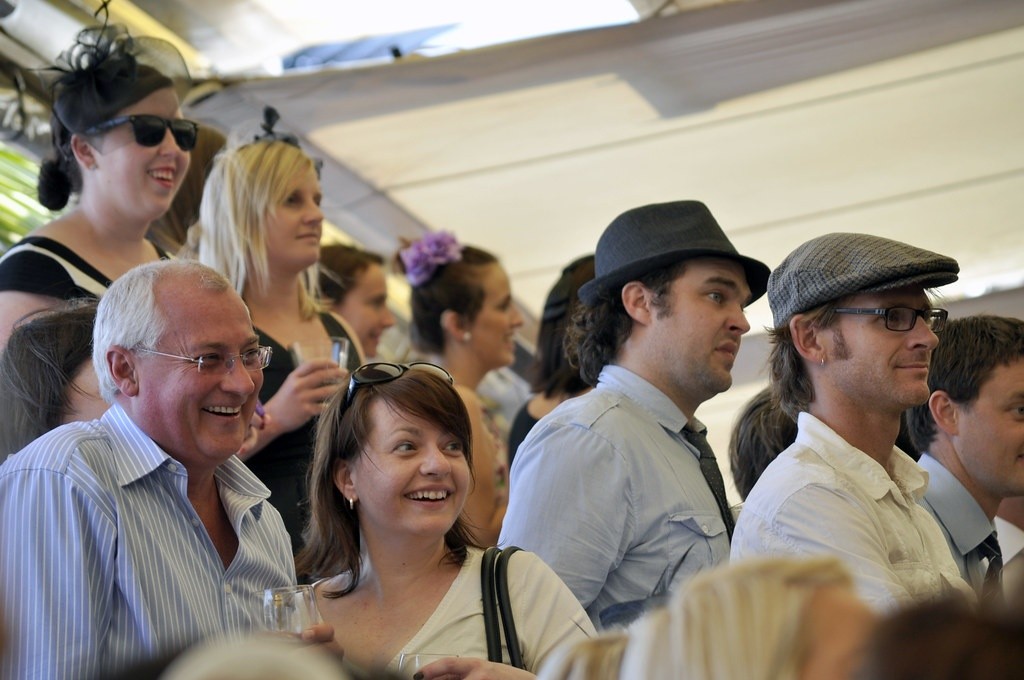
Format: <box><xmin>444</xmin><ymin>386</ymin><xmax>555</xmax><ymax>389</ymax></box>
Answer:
<box><xmin>768</xmin><ymin>233</ymin><xmax>960</xmax><ymax>330</ymax></box>
<box><xmin>577</xmin><ymin>201</ymin><xmax>771</xmax><ymax>308</ymax></box>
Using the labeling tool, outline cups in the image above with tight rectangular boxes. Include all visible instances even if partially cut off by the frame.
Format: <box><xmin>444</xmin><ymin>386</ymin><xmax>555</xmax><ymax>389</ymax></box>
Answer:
<box><xmin>248</xmin><ymin>585</ymin><xmax>319</xmax><ymax>637</ymax></box>
<box><xmin>289</xmin><ymin>335</ymin><xmax>351</xmax><ymax>404</ymax></box>
<box><xmin>396</xmin><ymin>652</ymin><xmax>460</xmax><ymax>680</ymax></box>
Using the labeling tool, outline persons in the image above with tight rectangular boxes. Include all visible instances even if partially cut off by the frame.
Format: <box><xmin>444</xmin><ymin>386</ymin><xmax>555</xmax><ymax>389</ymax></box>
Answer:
<box><xmin>0</xmin><ymin>23</ymin><xmax>1024</xmax><ymax>680</ymax></box>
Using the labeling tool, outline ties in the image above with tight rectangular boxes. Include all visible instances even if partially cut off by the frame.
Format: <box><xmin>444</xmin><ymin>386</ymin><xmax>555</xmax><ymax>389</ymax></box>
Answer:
<box><xmin>681</xmin><ymin>422</ymin><xmax>736</xmax><ymax>547</ymax></box>
<box><xmin>977</xmin><ymin>531</ymin><xmax>1003</xmax><ymax>596</ymax></box>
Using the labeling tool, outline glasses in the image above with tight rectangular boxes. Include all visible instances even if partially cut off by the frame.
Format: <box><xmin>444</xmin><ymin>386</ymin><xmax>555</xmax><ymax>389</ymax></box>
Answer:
<box><xmin>340</xmin><ymin>361</ymin><xmax>454</xmax><ymax>419</ymax></box>
<box><xmin>133</xmin><ymin>346</ymin><xmax>272</xmax><ymax>374</ymax></box>
<box><xmin>825</xmin><ymin>307</ymin><xmax>949</xmax><ymax>333</ymax></box>
<box><xmin>66</xmin><ymin>113</ymin><xmax>200</xmax><ymax>152</ymax></box>
